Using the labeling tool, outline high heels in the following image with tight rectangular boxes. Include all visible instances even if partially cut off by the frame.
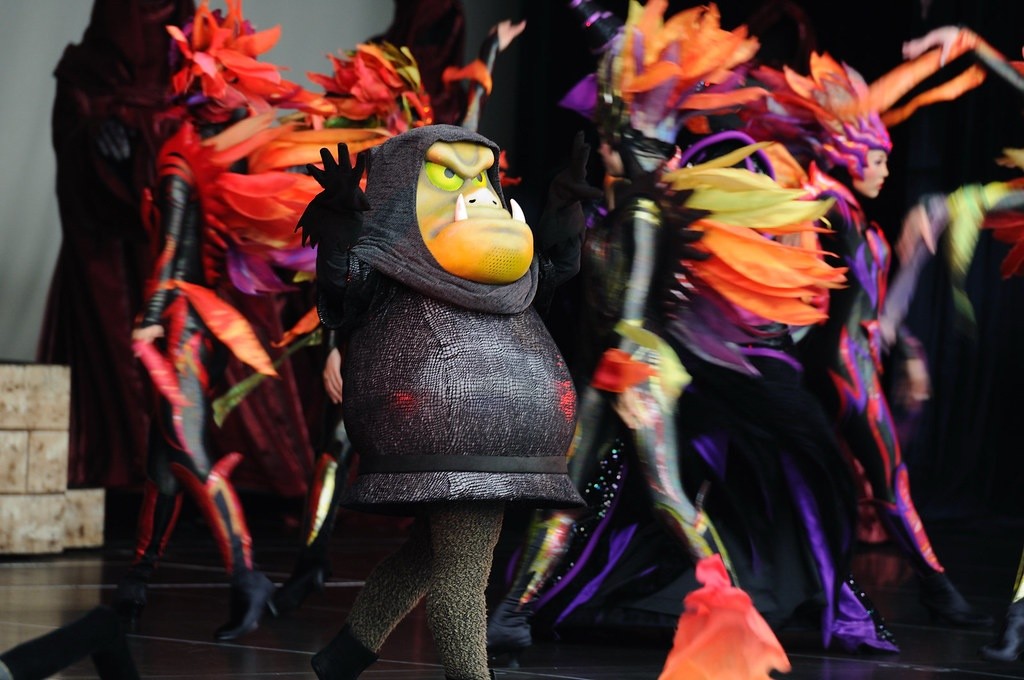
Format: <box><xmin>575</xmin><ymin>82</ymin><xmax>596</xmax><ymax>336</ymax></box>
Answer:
<box><xmin>977</xmin><ymin>613</ymin><xmax>1024</xmax><ymax>665</ymax></box>
<box><xmin>486</xmin><ymin>621</ymin><xmax>532</xmax><ymax>668</ymax></box>
<box><xmin>95</xmin><ymin>570</ymin><xmax>147</xmax><ymax>627</ymax></box>
<box><xmin>277</xmin><ymin>553</ymin><xmax>327</xmax><ymax>610</ymax></box>
<box><xmin>215</xmin><ymin>572</ymin><xmax>286</xmax><ymax>643</ymax></box>
<box><xmin>926</xmin><ymin>594</ymin><xmax>997</xmax><ymax>629</ymax></box>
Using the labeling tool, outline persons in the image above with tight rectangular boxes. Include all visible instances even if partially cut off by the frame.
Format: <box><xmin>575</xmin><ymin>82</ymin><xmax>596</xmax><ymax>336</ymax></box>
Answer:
<box><xmin>35</xmin><ymin>1</ymin><xmax>1024</xmax><ymax>680</ymax></box>
